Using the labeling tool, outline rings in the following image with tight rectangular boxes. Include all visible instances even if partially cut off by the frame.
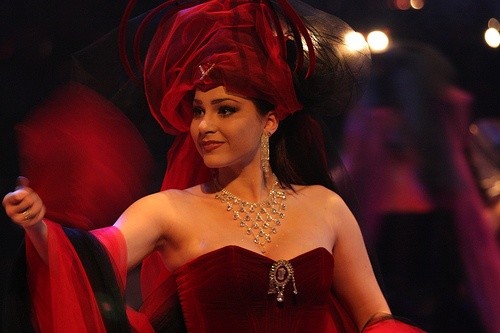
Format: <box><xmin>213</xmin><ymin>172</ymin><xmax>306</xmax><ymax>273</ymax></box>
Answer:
<box><xmin>23</xmin><ymin>210</ymin><xmax>31</xmax><ymax>219</ymax></box>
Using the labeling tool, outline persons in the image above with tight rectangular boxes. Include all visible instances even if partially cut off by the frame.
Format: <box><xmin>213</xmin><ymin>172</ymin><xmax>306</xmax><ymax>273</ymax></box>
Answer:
<box><xmin>2</xmin><ymin>1</ymin><xmax>425</xmax><ymax>333</ymax></box>
<box><xmin>330</xmin><ymin>47</ymin><xmax>487</xmax><ymax>333</ymax></box>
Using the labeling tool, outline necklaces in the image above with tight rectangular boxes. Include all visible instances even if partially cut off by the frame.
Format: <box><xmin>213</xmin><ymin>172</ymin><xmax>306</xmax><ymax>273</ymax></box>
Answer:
<box><xmin>208</xmin><ymin>173</ymin><xmax>286</xmax><ymax>254</ymax></box>
